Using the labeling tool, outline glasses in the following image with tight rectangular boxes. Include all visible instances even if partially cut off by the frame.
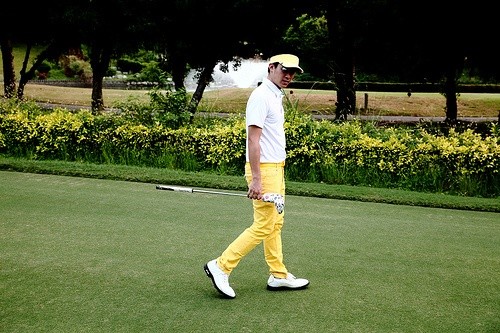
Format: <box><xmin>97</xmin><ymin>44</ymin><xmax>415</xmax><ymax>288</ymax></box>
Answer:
<box><xmin>280</xmin><ymin>67</ymin><xmax>298</xmax><ymax>74</ymax></box>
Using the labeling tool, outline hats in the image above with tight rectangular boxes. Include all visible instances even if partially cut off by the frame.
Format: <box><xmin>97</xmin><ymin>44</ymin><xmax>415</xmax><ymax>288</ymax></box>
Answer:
<box><xmin>269</xmin><ymin>53</ymin><xmax>304</xmax><ymax>74</ymax></box>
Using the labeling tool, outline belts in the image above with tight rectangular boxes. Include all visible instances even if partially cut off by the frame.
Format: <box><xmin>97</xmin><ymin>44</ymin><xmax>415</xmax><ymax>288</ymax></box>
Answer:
<box><xmin>246</xmin><ymin>160</ymin><xmax>285</xmax><ymax>166</ymax></box>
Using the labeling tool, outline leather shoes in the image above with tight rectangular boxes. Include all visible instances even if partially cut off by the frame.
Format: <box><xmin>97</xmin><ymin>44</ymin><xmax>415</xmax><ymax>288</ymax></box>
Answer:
<box><xmin>204</xmin><ymin>259</ymin><xmax>236</xmax><ymax>298</ymax></box>
<box><xmin>266</xmin><ymin>272</ymin><xmax>309</xmax><ymax>290</ymax></box>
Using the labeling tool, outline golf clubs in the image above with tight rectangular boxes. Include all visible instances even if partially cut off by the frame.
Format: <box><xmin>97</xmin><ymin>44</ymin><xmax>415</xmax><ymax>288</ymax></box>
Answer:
<box><xmin>156</xmin><ymin>185</ymin><xmax>284</xmax><ymax>214</ymax></box>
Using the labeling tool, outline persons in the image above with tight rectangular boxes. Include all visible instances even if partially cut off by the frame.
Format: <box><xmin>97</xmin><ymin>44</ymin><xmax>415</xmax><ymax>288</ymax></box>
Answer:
<box><xmin>205</xmin><ymin>43</ymin><xmax>310</xmax><ymax>298</ymax></box>
